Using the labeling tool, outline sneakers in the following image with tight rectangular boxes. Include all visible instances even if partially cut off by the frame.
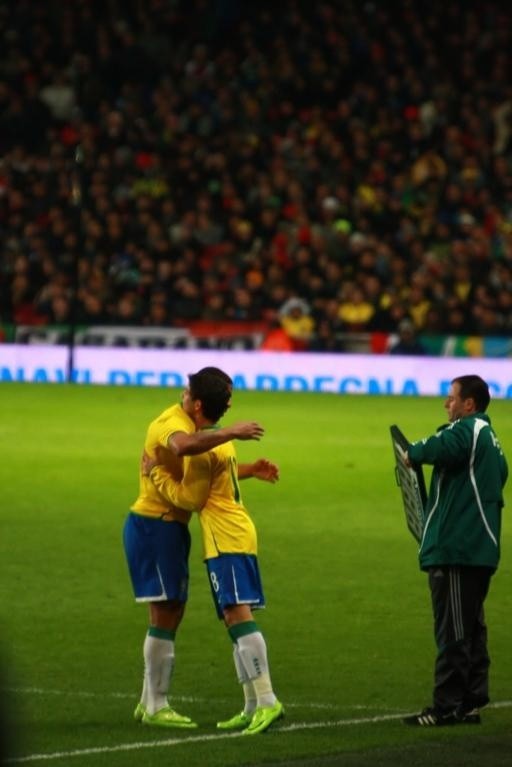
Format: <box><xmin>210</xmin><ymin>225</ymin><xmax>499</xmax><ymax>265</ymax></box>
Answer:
<box><xmin>133</xmin><ymin>702</ymin><xmax>198</xmax><ymax>728</ymax></box>
<box><xmin>216</xmin><ymin>698</ymin><xmax>286</xmax><ymax>736</ymax></box>
<box><xmin>401</xmin><ymin>704</ymin><xmax>482</xmax><ymax>727</ymax></box>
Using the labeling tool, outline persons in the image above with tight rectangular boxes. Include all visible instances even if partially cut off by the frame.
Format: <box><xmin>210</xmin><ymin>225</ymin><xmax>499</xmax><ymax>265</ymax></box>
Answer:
<box><xmin>124</xmin><ymin>369</ymin><xmax>286</xmax><ymax>736</ymax></box>
<box><xmin>0</xmin><ymin>1</ymin><xmax>510</xmax><ymax>357</ymax></box>
<box><xmin>402</xmin><ymin>375</ymin><xmax>508</xmax><ymax>727</ymax></box>
